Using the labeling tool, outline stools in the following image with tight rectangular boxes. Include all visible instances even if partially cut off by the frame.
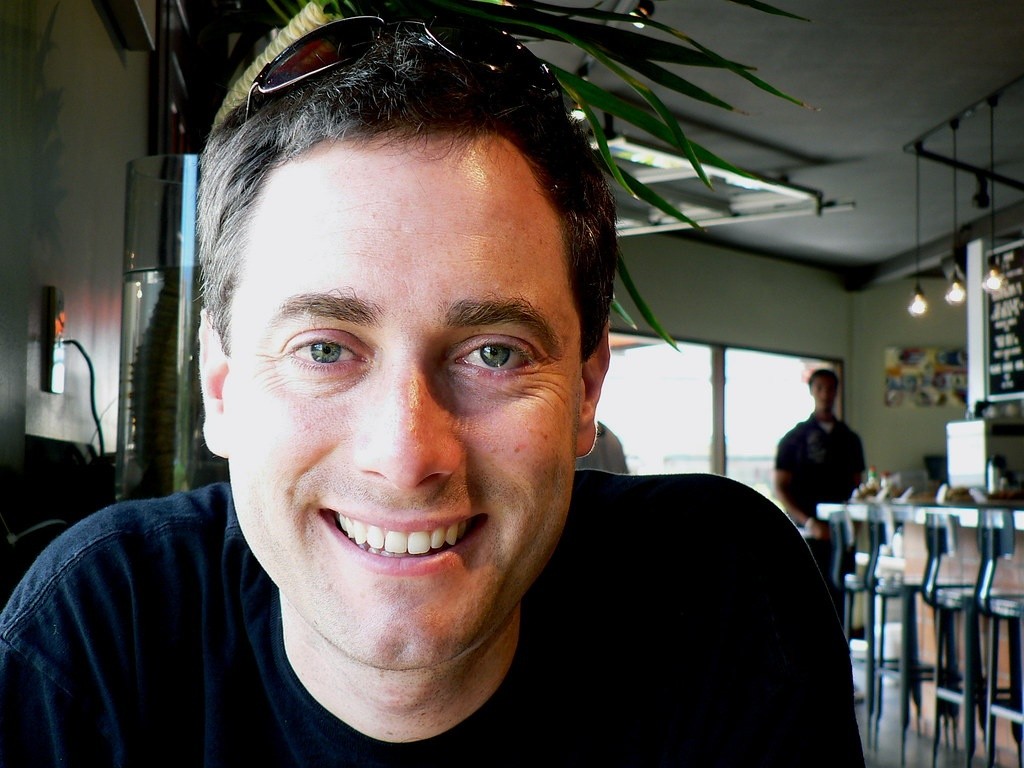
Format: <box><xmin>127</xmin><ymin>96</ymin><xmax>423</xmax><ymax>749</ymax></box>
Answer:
<box><xmin>862</xmin><ymin>502</ymin><xmax>974</xmax><ymax>768</ymax></box>
<box><xmin>970</xmin><ymin>505</ymin><xmax>1024</xmax><ymax>768</ymax></box>
<box><xmin>829</xmin><ymin>509</ymin><xmax>928</xmax><ymax>748</ymax></box>
<box><xmin>915</xmin><ymin>508</ymin><xmax>1022</xmax><ymax>768</ymax></box>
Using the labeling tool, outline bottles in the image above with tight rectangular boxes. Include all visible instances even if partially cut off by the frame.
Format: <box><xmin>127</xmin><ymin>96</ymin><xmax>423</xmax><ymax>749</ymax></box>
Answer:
<box><xmin>868</xmin><ymin>465</ymin><xmax>877</xmax><ymax>484</ymax></box>
<box><xmin>881</xmin><ymin>471</ymin><xmax>891</xmax><ymax>499</ymax></box>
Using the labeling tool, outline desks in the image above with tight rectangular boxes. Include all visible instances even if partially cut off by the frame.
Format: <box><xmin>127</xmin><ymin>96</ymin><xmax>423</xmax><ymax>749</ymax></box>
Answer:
<box><xmin>817</xmin><ymin>504</ymin><xmax>1024</xmax><ymax>768</ymax></box>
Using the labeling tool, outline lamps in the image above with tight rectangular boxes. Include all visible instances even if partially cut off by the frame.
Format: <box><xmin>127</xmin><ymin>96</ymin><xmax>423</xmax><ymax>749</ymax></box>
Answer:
<box><xmin>948</xmin><ymin>117</ymin><xmax>965</xmax><ymax>308</ymax></box>
<box><xmin>970</xmin><ymin>175</ymin><xmax>990</xmax><ymax>209</ymax></box>
<box><xmin>911</xmin><ymin>140</ymin><xmax>926</xmax><ymax>317</ymax></box>
<box><xmin>987</xmin><ymin>97</ymin><xmax>1004</xmax><ymax>291</ymax></box>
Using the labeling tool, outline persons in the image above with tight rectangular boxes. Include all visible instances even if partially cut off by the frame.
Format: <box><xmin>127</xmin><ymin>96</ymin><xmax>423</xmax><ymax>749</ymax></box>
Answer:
<box><xmin>573</xmin><ymin>419</ymin><xmax>628</xmax><ymax>477</ymax></box>
<box><xmin>776</xmin><ymin>368</ymin><xmax>868</xmax><ymax>708</ymax></box>
<box><xmin>1</xmin><ymin>18</ymin><xmax>866</xmax><ymax>764</ymax></box>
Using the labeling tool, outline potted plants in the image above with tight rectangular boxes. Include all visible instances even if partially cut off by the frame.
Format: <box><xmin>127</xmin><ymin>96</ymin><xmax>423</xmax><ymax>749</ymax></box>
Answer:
<box><xmin>119</xmin><ymin>0</ymin><xmax>826</xmax><ymax>493</ymax></box>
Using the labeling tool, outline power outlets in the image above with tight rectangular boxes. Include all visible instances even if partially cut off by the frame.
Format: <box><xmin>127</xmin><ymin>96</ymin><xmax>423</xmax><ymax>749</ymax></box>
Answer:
<box><xmin>44</xmin><ymin>287</ymin><xmax>68</xmax><ymax>390</ymax></box>
